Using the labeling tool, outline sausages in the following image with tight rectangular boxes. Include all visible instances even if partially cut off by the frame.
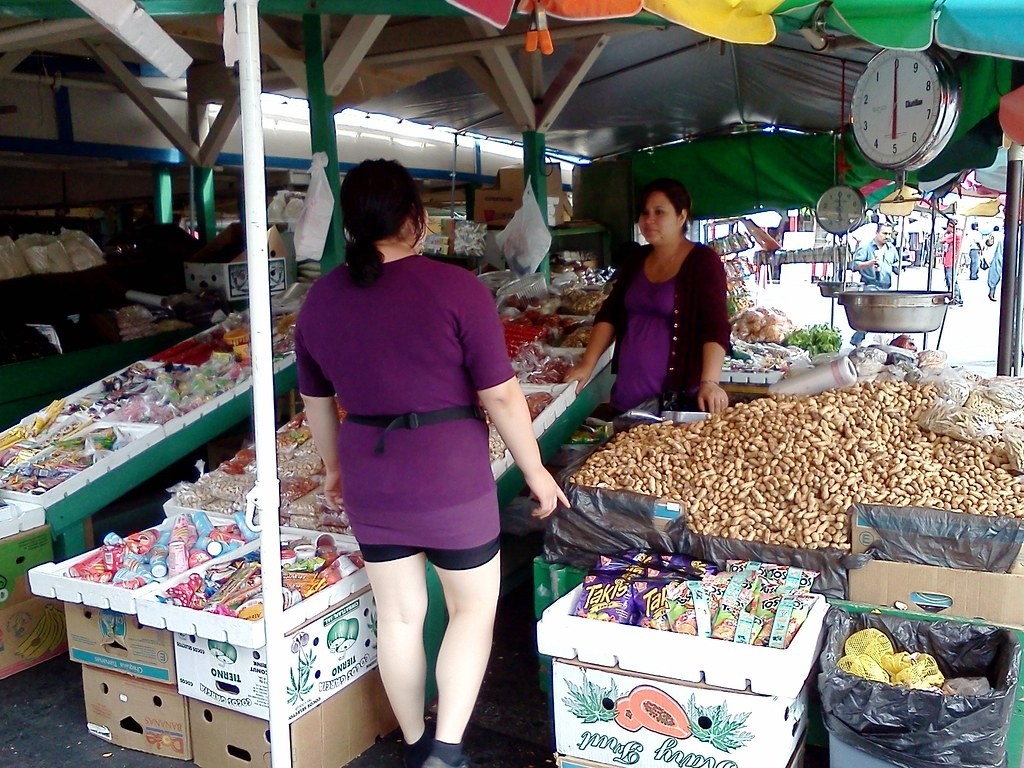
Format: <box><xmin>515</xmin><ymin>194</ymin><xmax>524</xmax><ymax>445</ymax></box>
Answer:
<box><xmin>502</xmin><ymin>323</ymin><xmax>549</xmax><ymax>359</ymax></box>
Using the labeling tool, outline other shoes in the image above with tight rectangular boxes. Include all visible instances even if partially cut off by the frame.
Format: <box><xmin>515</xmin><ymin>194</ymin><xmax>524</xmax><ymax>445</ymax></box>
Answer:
<box><xmin>403</xmin><ymin>743</ymin><xmax>481</xmax><ymax>768</ymax></box>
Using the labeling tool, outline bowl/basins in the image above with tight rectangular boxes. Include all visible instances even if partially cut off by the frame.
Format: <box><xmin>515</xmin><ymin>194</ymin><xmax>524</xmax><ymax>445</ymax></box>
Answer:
<box><xmin>426</xmin><ymin>235</ymin><xmax>449</xmax><ymax>254</ymax></box>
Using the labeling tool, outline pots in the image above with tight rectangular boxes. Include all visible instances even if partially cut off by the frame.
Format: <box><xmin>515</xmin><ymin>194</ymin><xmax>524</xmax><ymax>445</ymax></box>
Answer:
<box><xmin>811</xmin><ymin>280</ymin><xmax>865</xmax><ymax>299</ymax></box>
<box><xmin>835</xmin><ymin>280</ymin><xmax>956</xmax><ymax>334</ymax></box>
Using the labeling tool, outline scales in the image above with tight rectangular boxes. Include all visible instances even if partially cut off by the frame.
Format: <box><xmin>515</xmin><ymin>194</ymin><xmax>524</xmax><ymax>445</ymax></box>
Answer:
<box><xmin>832</xmin><ymin>47</ymin><xmax>963</xmax><ymax>335</ymax></box>
<box><xmin>815</xmin><ymin>184</ymin><xmax>868</xmax><ymax>299</ymax></box>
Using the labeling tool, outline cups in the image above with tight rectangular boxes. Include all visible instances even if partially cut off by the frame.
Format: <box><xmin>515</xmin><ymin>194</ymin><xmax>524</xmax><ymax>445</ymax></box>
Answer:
<box><xmin>67</xmin><ymin>511</ymin><xmax>359</xmax><ymax>586</ymax></box>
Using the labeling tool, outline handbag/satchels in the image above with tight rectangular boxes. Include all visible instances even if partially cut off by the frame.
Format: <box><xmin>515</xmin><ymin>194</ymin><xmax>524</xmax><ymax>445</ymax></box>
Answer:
<box><xmin>986</xmin><ymin>232</ymin><xmax>994</xmax><ymax>245</ymax></box>
<box><xmin>495</xmin><ymin>175</ymin><xmax>551</xmax><ymax>278</ymax></box>
<box><xmin>980</xmin><ymin>257</ymin><xmax>990</xmax><ymax>270</ymax></box>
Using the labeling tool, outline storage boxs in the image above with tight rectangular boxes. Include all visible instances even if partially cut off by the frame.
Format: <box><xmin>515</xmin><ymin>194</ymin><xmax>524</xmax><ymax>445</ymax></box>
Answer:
<box><xmin>0</xmin><ymin>525</ymin><xmax>401</xmax><ymax>768</ymax></box>
<box><xmin>846</xmin><ymin>503</ymin><xmax>1023</xmax><ymax>629</ymax></box>
<box><xmin>535</xmin><ymin>579</ymin><xmax>832</xmax><ymax>768</ymax></box>
<box><xmin>476</xmin><ymin>161</ymin><xmax>615</xmax><ymax>227</ymax></box>
<box><xmin>187</xmin><ymin>220</ymin><xmax>291</xmax><ymax>302</ymax></box>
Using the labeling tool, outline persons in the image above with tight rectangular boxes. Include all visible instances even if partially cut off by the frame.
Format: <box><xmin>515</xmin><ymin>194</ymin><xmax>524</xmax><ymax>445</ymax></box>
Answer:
<box><xmin>850</xmin><ymin>222</ymin><xmax>905</xmax><ymax>347</ymax></box>
<box><xmin>923</xmin><ymin>235</ymin><xmax>931</xmax><ymax>266</ymax></box>
<box><xmin>295</xmin><ymin>158</ymin><xmax>570</xmax><ymax>768</ymax></box>
<box><xmin>564</xmin><ymin>177</ymin><xmax>732</xmax><ymax>414</ymax></box>
<box><xmin>969</xmin><ymin>223</ymin><xmax>984</xmax><ymax>280</ymax></box>
<box><xmin>988</xmin><ymin>217</ymin><xmax>1024</xmax><ymax>367</ymax></box>
<box><xmin>940</xmin><ymin>218</ymin><xmax>963</xmax><ymax>308</ymax></box>
<box><xmin>101</xmin><ymin>165</ymin><xmax>187</xmax><ymax>253</ymax></box>
<box><xmin>985</xmin><ymin>225</ymin><xmax>1003</xmax><ymax>264</ymax></box>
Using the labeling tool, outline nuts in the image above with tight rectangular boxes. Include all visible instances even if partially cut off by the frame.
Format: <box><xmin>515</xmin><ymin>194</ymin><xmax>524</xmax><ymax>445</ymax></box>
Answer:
<box><xmin>569</xmin><ymin>380</ymin><xmax>1023</xmax><ymax>552</ymax></box>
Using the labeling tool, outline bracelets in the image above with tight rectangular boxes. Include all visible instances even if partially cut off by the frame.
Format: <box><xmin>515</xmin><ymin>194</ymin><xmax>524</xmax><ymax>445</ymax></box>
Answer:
<box><xmin>700</xmin><ymin>380</ymin><xmax>718</xmax><ymax>384</ymax></box>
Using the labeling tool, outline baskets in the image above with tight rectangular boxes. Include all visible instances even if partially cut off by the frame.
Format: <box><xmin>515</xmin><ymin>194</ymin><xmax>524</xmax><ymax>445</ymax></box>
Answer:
<box><xmin>497</xmin><ymin>273</ymin><xmax>548</xmax><ymax>310</ymax></box>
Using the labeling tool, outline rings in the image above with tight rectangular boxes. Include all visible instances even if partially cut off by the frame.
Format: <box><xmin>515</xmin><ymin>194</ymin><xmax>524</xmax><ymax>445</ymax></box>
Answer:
<box><xmin>721</xmin><ymin>400</ymin><xmax>724</xmax><ymax>402</ymax></box>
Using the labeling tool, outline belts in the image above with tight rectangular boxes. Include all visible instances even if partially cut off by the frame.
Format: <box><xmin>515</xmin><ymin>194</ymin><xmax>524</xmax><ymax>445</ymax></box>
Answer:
<box><xmin>345</xmin><ymin>404</ymin><xmax>485</xmax><ymax>455</ymax></box>
